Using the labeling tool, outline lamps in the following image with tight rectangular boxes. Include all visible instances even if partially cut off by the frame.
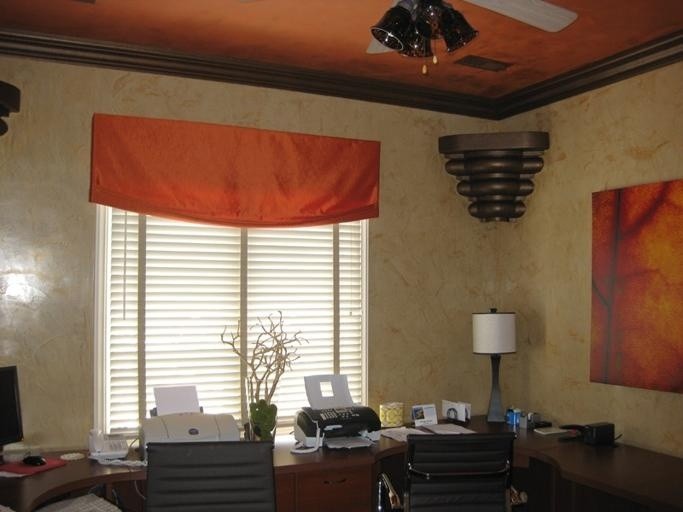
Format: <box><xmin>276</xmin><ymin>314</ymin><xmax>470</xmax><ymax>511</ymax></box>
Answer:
<box><xmin>469</xmin><ymin>303</ymin><xmax>521</xmax><ymax>423</ymax></box>
<box><xmin>369</xmin><ymin>0</ymin><xmax>476</xmax><ymax>75</ymax></box>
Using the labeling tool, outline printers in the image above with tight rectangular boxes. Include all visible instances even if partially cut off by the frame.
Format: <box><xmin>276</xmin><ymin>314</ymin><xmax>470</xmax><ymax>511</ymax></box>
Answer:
<box><xmin>291</xmin><ymin>403</ymin><xmax>381</xmax><ymax>454</ymax></box>
<box><xmin>139</xmin><ymin>407</ymin><xmax>239</xmax><ymax>462</ymax></box>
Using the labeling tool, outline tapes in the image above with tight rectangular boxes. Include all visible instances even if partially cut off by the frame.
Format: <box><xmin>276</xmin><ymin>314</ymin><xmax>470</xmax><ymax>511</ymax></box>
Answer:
<box><xmin>527</xmin><ymin>412</ymin><xmax>534</xmax><ymax>420</ymax></box>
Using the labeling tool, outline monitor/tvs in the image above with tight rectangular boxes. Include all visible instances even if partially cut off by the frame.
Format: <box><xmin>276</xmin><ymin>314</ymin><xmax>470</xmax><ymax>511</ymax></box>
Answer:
<box><xmin>0</xmin><ymin>366</ymin><xmax>24</xmax><ymax>465</ymax></box>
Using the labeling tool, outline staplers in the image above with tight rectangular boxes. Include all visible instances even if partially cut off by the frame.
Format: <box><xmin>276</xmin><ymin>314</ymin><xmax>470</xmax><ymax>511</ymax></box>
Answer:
<box><xmin>558</xmin><ymin>424</ymin><xmax>585</xmax><ymax>442</ymax></box>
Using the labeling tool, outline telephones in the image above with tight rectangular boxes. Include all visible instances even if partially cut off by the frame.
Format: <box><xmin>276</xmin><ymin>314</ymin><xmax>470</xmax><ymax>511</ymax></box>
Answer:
<box><xmin>88</xmin><ymin>429</ymin><xmax>128</xmax><ymax>460</ymax></box>
<box><xmin>294</xmin><ymin>407</ymin><xmax>324</xmax><ymax>447</ymax></box>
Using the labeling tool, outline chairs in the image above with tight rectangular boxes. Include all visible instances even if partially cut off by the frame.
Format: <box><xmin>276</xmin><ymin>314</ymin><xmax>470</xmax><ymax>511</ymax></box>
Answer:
<box><xmin>139</xmin><ymin>436</ymin><xmax>279</xmax><ymax>512</ymax></box>
<box><xmin>370</xmin><ymin>429</ymin><xmax>532</xmax><ymax>511</ymax></box>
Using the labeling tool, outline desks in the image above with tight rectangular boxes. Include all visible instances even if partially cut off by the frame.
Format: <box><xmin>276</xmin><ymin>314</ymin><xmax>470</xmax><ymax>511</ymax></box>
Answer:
<box><xmin>375</xmin><ymin>413</ymin><xmax>586</xmax><ymax>465</ymax></box>
<box><xmin>1</xmin><ymin>432</ymin><xmax>376</xmax><ymax>511</ymax></box>
<box><xmin>513</xmin><ymin>419</ymin><xmax>682</xmax><ymax>511</ymax></box>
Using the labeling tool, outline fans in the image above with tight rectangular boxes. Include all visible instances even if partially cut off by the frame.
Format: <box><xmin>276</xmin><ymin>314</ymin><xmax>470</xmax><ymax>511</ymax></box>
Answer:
<box><xmin>363</xmin><ymin>0</ymin><xmax>579</xmax><ymax>56</ymax></box>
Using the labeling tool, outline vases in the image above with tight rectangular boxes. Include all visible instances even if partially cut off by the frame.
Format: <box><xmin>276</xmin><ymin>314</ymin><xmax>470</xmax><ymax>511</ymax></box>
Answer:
<box><xmin>248</xmin><ymin>422</ymin><xmax>276</xmax><ymax>449</ymax></box>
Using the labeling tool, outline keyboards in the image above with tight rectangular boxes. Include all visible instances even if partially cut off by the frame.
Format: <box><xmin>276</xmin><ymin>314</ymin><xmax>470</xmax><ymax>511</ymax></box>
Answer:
<box><xmin>58</xmin><ymin>493</ymin><xmax>122</xmax><ymax>512</ymax></box>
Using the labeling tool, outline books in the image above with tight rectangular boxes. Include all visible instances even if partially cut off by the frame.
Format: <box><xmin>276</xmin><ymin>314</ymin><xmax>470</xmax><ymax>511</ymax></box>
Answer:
<box><xmin>534</xmin><ymin>426</ymin><xmax>568</xmax><ymax>436</ymax></box>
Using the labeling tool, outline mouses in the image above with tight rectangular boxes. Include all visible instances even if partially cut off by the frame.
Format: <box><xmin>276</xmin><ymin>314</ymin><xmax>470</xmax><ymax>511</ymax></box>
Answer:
<box><xmin>23</xmin><ymin>456</ymin><xmax>47</xmax><ymax>466</ymax></box>
